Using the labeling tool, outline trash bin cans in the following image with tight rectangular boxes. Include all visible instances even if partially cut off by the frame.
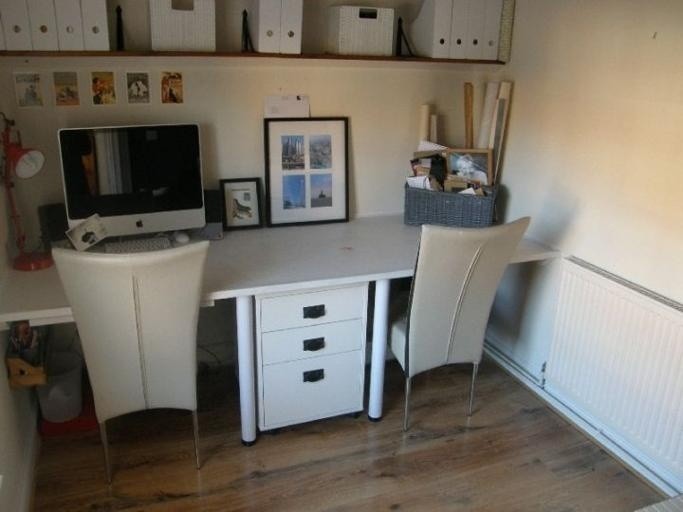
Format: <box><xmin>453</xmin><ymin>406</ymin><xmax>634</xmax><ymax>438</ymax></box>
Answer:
<box><xmin>36</xmin><ymin>350</ymin><xmax>84</xmax><ymax>424</ymax></box>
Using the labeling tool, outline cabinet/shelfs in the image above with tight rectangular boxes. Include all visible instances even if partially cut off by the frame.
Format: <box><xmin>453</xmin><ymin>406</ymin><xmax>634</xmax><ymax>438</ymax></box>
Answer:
<box><xmin>256</xmin><ymin>284</ymin><xmax>370</xmax><ymax>432</ymax></box>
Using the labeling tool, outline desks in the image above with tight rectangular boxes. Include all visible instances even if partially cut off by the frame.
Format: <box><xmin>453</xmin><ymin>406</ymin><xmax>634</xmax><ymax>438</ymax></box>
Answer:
<box><xmin>1</xmin><ymin>217</ymin><xmax>555</xmax><ymax>446</ymax></box>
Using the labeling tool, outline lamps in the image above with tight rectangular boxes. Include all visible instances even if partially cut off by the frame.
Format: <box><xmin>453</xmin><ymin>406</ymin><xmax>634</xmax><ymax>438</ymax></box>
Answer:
<box><xmin>1</xmin><ymin>112</ymin><xmax>54</xmax><ymax>272</ymax></box>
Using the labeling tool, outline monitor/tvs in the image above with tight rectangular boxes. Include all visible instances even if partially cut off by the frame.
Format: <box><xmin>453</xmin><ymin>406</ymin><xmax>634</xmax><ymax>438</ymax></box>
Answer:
<box><xmin>57</xmin><ymin>124</ymin><xmax>207</xmax><ymax>237</ymax></box>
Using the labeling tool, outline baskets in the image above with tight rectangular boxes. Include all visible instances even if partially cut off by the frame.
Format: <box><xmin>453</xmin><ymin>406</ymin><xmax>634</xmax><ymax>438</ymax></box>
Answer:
<box><xmin>404</xmin><ymin>182</ymin><xmax>497</xmax><ymax>227</ymax></box>
<box><xmin>327</xmin><ymin>6</ymin><xmax>395</xmax><ymax>57</ymax></box>
<box><xmin>148</xmin><ymin>0</ymin><xmax>216</xmax><ymax>53</ymax></box>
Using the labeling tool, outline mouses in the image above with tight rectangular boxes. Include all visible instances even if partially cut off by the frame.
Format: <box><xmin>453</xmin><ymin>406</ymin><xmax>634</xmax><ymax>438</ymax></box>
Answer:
<box><xmin>174</xmin><ymin>233</ymin><xmax>189</xmax><ymax>243</ymax></box>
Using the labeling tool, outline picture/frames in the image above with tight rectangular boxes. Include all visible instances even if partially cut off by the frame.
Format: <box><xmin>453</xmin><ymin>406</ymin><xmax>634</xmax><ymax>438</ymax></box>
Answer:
<box><xmin>264</xmin><ymin>116</ymin><xmax>349</xmax><ymax>227</ymax></box>
<box><xmin>446</xmin><ymin>148</ymin><xmax>493</xmax><ymax>187</ymax></box>
<box><xmin>219</xmin><ymin>177</ymin><xmax>264</xmax><ymax>231</ymax></box>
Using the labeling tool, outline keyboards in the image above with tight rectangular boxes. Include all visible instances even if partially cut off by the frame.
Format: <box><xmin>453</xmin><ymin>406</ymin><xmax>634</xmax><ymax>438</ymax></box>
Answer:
<box><xmin>104</xmin><ymin>238</ymin><xmax>172</xmax><ymax>254</ymax></box>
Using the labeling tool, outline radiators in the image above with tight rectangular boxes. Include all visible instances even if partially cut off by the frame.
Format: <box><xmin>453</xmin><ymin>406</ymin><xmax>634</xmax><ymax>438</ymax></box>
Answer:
<box><xmin>539</xmin><ymin>255</ymin><xmax>683</xmax><ymax>487</ymax></box>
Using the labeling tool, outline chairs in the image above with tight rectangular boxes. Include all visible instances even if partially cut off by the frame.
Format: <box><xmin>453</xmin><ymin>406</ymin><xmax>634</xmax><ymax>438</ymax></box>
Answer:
<box><xmin>51</xmin><ymin>239</ymin><xmax>212</xmax><ymax>484</ymax></box>
<box><xmin>389</xmin><ymin>218</ymin><xmax>531</xmax><ymax>432</ymax></box>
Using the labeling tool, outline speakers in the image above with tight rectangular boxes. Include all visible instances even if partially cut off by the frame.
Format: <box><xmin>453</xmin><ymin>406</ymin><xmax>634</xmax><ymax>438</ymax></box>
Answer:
<box><xmin>38</xmin><ymin>203</ymin><xmax>68</xmax><ymax>249</ymax></box>
<box><xmin>204</xmin><ymin>190</ymin><xmax>223</xmax><ymax>239</ymax></box>
